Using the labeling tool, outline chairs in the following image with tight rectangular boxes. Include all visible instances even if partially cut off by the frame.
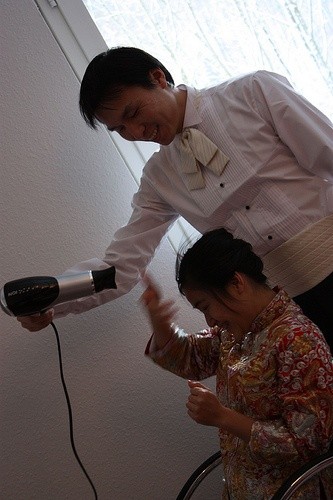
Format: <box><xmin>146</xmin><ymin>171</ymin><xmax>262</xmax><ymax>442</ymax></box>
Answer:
<box><xmin>174</xmin><ymin>448</ymin><xmax>333</xmax><ymax>500</ymax></box>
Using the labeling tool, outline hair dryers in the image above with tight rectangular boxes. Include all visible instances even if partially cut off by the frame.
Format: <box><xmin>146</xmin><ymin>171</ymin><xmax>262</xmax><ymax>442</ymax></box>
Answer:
<box><xmin>0</xmin><ymin>266</ymin><xmax>118</xmax><ymax>317</ymax></box>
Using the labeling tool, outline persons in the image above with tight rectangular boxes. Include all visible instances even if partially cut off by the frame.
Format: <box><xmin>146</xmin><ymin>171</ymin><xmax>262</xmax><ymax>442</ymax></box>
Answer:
<box><xmin>16</xmin><ymin>45</ymin><xmax>333</xmax><ymax>356</ymax></box>
<box><xmin>141</xmin><ymin>225</ymin><xmax>333</xmax><ymax>500</ymax></box>
